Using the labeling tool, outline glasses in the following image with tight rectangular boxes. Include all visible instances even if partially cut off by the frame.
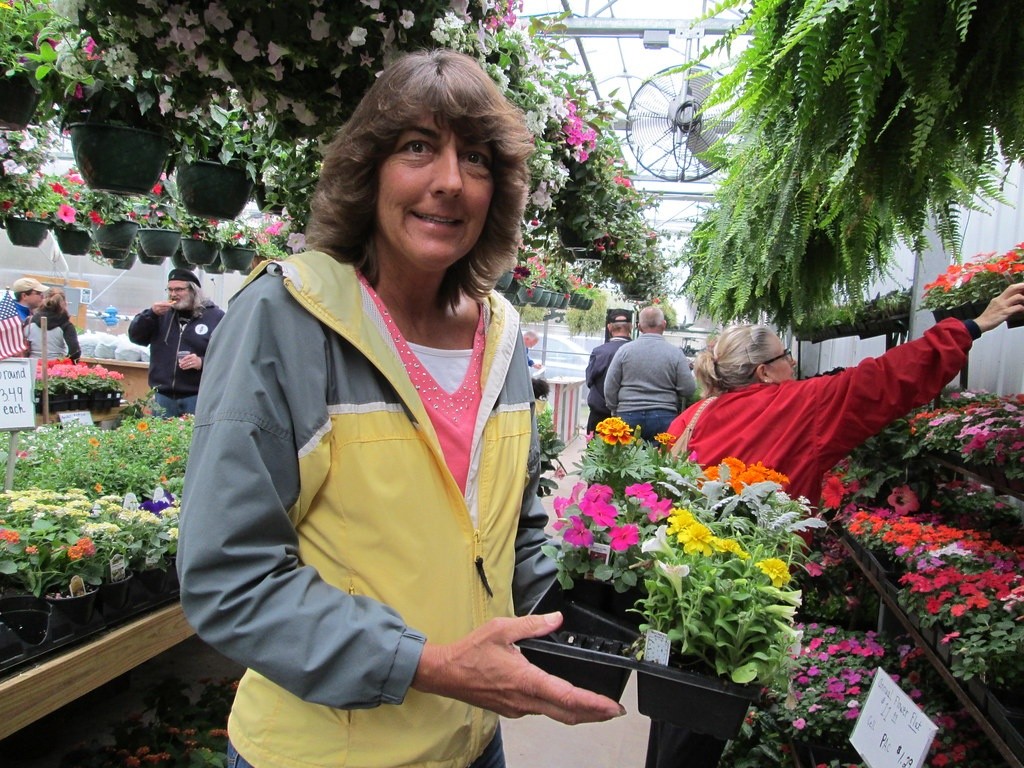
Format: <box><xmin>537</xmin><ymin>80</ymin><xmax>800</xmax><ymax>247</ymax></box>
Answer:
<box><xmin>747</xmin><ymin>348</ymin><xmax>793</xmax><ymax>379</ymax></box>
<box><xmin>164</xmin><ymin>286</ymin><xmax>191</xmax><ymax>294</ymax></box>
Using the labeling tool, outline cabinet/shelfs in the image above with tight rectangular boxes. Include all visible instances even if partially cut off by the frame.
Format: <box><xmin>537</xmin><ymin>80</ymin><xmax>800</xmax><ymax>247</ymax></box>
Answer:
<box><xmin>796</xmin><ymin>314</ymin><xmax>1024</xmax><ymax>768</ymax></box>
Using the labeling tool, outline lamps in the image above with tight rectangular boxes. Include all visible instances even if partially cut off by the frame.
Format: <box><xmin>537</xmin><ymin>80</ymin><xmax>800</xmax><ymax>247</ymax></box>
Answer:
<box><xmin>642</xmin><ymin>29</ymin><xmax>669</xmax><ymax>50</ymax></box>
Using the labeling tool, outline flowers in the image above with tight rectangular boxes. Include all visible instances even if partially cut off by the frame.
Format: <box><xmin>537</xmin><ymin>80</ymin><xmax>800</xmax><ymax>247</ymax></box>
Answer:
<box><xmin>0</xmin><ymin>0</ymin><xmax>1024</xmax><ymax>768</ymax></box>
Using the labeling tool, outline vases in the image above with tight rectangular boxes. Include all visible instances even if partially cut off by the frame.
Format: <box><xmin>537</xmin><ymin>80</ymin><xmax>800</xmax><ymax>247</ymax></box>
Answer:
<box><xmin>531</xmin><ymin>290</ymin><xmax>551</xmax><ymax>308</ymax></box>
<box><xmin>569</xmin><ymin>294</ymin><xmax>580</xmax><ymax>306</ymax></box>
<box><xmin>111</xmin><ymin>391</ymin><xmax>122</xmax><ymax>408</ymax></box>
<box><xmin>137</xmin><ymin>229</ymin><xmax>183</xmax><ymax>258</ymax></box>
<box><xmin>181</xmin><ymin>237</ymin><xmax>223</xmax><ymax>266</ymax></box>
<box><xmin>69</xmin><ymin>392</ymin><xmax>91</xmax><ymax>410</ymax></box>
<box><xmin>854</xmin><ymin>543</ymin><xmax>864</xmax><ymax>561</ymax></box>
<box><xmin>967</xmin><ymin>460</ymin><xmax>986</xmax><ymax>476</ymax></box>
<box><xmin>495</xmin><ymin>270</ymin><xmax>515</xmax><ymax>290</ymax></box>
<box><xmin>89</xmin><ymin>391</ymin><xmax>112</xmax><ymax>411</ymax></box>
<box><xmin>171</xmin><ymin>247</ymin><xmax>198</xmax><ymax>272</ymax></box>
<box><xmin>137</xmin><ymin>247</ymin><xmax>167</xmax><ymax>266</ymax></box>
<box><xmin>4</xmin><ymin>217</ymin><xmax>51</xmax><ymax>248</ymax></box>
<box><xmin>129</xmin><ymin>558</ymin><xmax>169</xmax><ymax>594</ymax></box>
<box><xmin>511</xmin><ymin>566</ymin><xmax>764</xmax><ymax>743</ymax></box>
<box><xmin>503</xmin><ymin>292</ymin><xmax>515</xmax><ymax>304</ymax></box>
<box><xmin>254</xmin><ymin>185</ymin><xmax>287</xmax><ymax>216</ymax></box>
<box><xmin>998</xmin><ymin>714</ymin><xmax>1024</xmax><ymax>767</ymax></box>
<box><xmin>968</xmin><ymin>676</ymin><xmax>988</xmax><ymax>714</ymax></box>
<box><xmin>576</xmin><ymin>297</ymin><xmax>584</xmax><ymax>308</ymax></box>
<box><xmin>584</xmin><ymin>300</ymin><xmax>593</xmax><ymax>310</ymax></box>
<box><xmin>630</xmin><ymin>295</ymin><xmax>645</xmax><ymax>301</ymax></box>
<box><xmin>555</xmin><ymin>294</ymin><xmax>565</xmax><ymax>308</ymax></box>
<box><xmin>204</xmin><ymin>253</ymin><xmax>225</xmax><ymax>274</ymax></box>
<box><xmin>517</xmin><ymin>284</ymin><xmax>544</xmax><ymax>304</ymax></box>
<box><xmin>907</xmin><ymin>609</ymin><xmax>922</xmax><ymax>629</ymax></box>
<box><xmin>504</xmin><ymin>278</ymin><xmax>518</xmax><ymax>294</ymax></box>
<box><xmin>63</xmin><ymin>122</ymin><xmax>175</xmax><ymax>198</ymax></box>
<box><xmin>92</xmin><ymin>219</ymin><xmax>140</xmax><ymax>251</ymax></box>
<box><xmin>622</xmin><ymin>282</ymin><xmax>640</xmax><ymax>296</ymax></box>
<box><xmin>512</xmin><ymin>295</ymin><xmax>526</xmax><ymax>307</ymax></box>
<box><xmin>111</xmin><ymin>253</ymin><xmax>138</xmax><ymax>270</ymax></box>
<box><xmin>55</xmin><ymin>225</ymin><xmax>92</xmax><ymax>256</ymax></box>
<box><xmin>946</xmin><ymin>451</ymin><xmax>962</xmax><ymax>465</ymax></box>
<box><xmin>219</xmin><ymin>245</ymin><xmax>255</xmax><ymax>271</ymax></box>
<box><xmin>880</xmin><ymin>576</ymin><xmax>901</xmax><ymax>603</ymax></box>
<box><xmin>919</xmin><ymin>619</ymin><xmax>936</xmax><ymax>648</ymax></box>
<box><xmin>1008</xmin><ymin>477</ymin><xmax>1024</xmax><ymax>494</ymax></box>
<box><xmin>560</xmin><ymin>297</ymin><xmax>569</xmax><ymax>308</ymax></box>
<box><xmin>225</xmin><ymin>267</ymin><xmax>235</xmax><ymax>274</ymax></box>
<box><xmin>558</xmin><ymin>205</ymin><xmax>594</xmax><ymax>251</ymax></box>
<box><xmin>863</xmin><ymin>548</ymin><xmax>899</xmax><ymax>580</ymax></box>
<box><xmin>36</xmin><ymin>394</ymin><xmax>53</xmax><ymax>415</ymax></box>
<box><xmin>48</xmin><ymin>393</ymin><xmax>71</xmax><ymax>410</ymax></box>
<box><xmin>572</xmin><ymin>249</ymin><xmax>607</xmax><ymax>261</ymax></box>
<box><xmin>582</xmin><ymin>299</ymin><xmax>588</xmax><ymax>310</ymax></box>
<box><xmin>0</xmin><ymin>73</ymin><xmax>41</xmax><ymax>132</ymax></box>
<box><xmin>547</xmin><ymin>292</ymin><xmax>559</xmax><ymax>307</ymax></box>
<box><xmin>1006</xmin><ymin>303</ymin><xmax>1024</xmax><ymax>330</ymax></box>
<box><xmin>176</xmin><ymin>158</ymin><xmax>255</xmax><ymax>222</ymax></box>
<box><xmin>239</xmin><ymin>268</ymin><xmax>252</xmax><ymax>276</ymax></box>
<box><xmin>934</xmin><ymin>631</ymin><xmax>951</xmax><ymax>667</ymax></box>
<box><xmin>987</xmin><ymin>690</ymin><xmax>1024</xmax><ymax>729</ymax></box>
<box><xmin>45</xmin><ymin>580</ymin><xmax>101</xmax><ymax>626</ymax></box>
<box><xmin>97</xmin><ymin>569</ymin><xmax>133</xmax><ymax>610</ymax></box>
<box><xmin>100</xmin><ymin>248</ymin><xmax>130</xmax><ymax>261</ymax></box>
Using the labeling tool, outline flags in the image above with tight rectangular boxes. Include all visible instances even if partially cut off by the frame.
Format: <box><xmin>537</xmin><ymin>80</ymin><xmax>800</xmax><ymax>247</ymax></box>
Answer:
<box><xmin>0</xmin><ymin>290</ymin><xmax>29</xmax><ymax>363</ymax></box>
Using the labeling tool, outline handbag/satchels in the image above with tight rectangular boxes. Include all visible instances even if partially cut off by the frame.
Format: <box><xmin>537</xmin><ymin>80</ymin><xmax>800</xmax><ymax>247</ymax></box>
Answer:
<box><xmin>12</xmin><ymin>315</ymin><xmax>33</xmax><ymax>357</ymax></box>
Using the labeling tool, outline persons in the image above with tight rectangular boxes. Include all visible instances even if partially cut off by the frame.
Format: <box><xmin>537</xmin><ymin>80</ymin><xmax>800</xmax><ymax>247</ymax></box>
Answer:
<box><xmin>11</xmin><ymin>277</ymin><xmax>50</xmax><ymax>323</ymax></box>
<box><xmin>173</xmin><ymin>50</ymin><xmax>625</xmax><ymax>768</ymax></box>
<box><xmin>584</xmin><ymin>309</ymin><xmax>633</xmax><ymax>442</ymax></box>
<box><xmin>523</xmin><ymin>330</ymin><xmax>542</xmax><ymax>370</ymax></box>
<box><xmin>23</xmin><ymin>287</ymin><xmax>82</xmax><ymax>364</ymax></box>
<box><xmin>604</xmin><ymin>305</ymin><xmax>696</xmax><ymax>454</ymax></box>
<box><xmin>128</xmin><ymin>269</ymin><xmax>226</xmax><ymax>421</ymax></box>
<box><xmin>635</xmin><ymin>282</ymin><xmax>1024</xmax><ymax>768</ymax></box>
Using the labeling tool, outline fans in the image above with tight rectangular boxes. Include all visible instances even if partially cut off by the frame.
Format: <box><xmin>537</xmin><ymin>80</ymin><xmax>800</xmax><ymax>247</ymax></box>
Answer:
<box><xmin>624</xmin><ymin>28</ymin><xmax>745</xmax><ymax>183</ymax></box>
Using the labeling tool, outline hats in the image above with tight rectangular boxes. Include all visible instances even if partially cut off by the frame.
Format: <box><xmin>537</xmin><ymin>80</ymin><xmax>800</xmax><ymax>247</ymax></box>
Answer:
<box><xmin>168</xmin><ymin>269</ymin><xmax>201</xmax><ymax>288</ymax></box>
<box><xmin>13</xmin><ymin>278</ymin><xmax>50</xmax><ymax>293</ymax></box>
<box><xmin>607</xmin><ymin>308</ymin><xmax>632</xmax><ymax>323</ymax></box>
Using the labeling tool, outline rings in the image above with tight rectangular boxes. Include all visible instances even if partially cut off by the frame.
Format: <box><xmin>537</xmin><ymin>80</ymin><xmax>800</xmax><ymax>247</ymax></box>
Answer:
<box><xmin>190</xmin><ymin>362</ymin><xmax>191</xmax><ymax>365</ymax></box>
